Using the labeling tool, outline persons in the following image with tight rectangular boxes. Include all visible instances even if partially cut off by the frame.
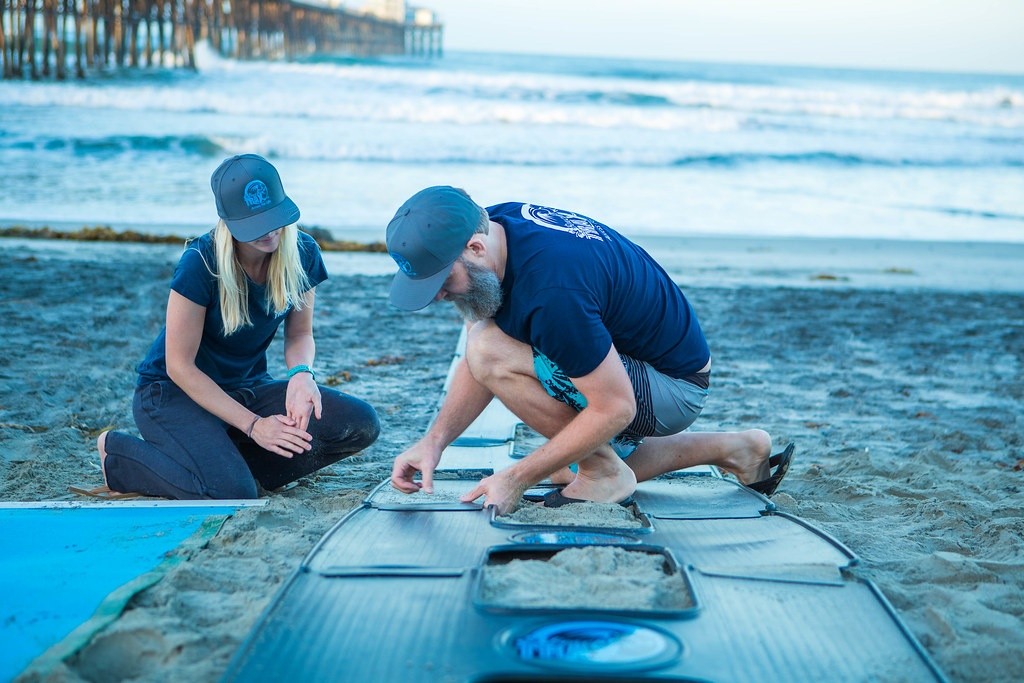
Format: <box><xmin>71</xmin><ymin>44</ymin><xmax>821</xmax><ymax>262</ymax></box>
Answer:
<box><xmin>386</xmin><ymin>185</ymin><xmax>795</xmax><ymax>516</ymax></box>
<box><xmin>97</xmin><ymin>154</ymin><xmax>380</xmax><ymax>500</ymax></box>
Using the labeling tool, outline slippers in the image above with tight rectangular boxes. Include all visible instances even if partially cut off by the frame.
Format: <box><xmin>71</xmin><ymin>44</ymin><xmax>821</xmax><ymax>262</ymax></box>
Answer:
<box><xmin>272</xmin><ymin>481</ymin><xmax>299</xmax><ymax>493</ymax></box>
<box><xmin>746</xmin><ymin>441</ymin><xmax>797</xmax><ymax>498</ymax></box>
<box><xmin>543</xmin><ymin>487</ymin><xmax>634</xmax><ymax>510</ymax></box>
<box><xmin>68</xmin><ymin>483</ymin><xmax>139</xmax><ymax>500</ymax></box>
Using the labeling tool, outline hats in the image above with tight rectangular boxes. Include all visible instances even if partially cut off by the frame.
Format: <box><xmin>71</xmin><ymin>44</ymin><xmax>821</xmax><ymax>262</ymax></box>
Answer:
<box><xmin>388</xmin><ymin>184</ymin><xmax>483</xmax><ymax>312</ymax></box>
<box><xmin>210</xmin><ymin>154</ymin><xmax>301</xmax><ymax>243</ymax></box>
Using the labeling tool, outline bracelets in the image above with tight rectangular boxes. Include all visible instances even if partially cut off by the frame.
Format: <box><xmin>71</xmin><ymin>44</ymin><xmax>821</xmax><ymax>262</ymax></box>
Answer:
<box><xmin>247</xmin><ymin>416</ymin><xmax>259</xmax><ymax>437</ymax></box>
<box><xmin>287</xmin><ymin>365</ymin><xmax>315</xmax><ymax>379</ymax></box>
